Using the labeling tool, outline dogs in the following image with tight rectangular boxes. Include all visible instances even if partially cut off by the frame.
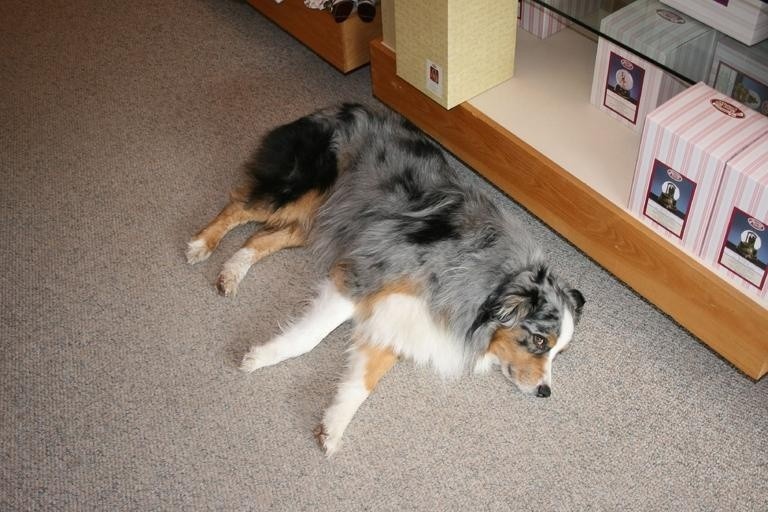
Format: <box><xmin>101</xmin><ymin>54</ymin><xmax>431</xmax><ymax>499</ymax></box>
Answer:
<box><xmin>184</xmin><ymin>101</ymin><xmax>587</xmax><ymax>459</ymax></box>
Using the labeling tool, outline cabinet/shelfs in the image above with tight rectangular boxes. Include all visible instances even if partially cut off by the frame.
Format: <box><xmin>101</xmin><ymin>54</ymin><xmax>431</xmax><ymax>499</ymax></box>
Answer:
<box><xmin>249</xmin><ymin>0</ymin><xmax>381</xmax><ymax>73</ymax></box>
<box><xmin>369</xmin><ymin>0</ymin><xmax>768</xmax><ymax>381</ymax></box>
